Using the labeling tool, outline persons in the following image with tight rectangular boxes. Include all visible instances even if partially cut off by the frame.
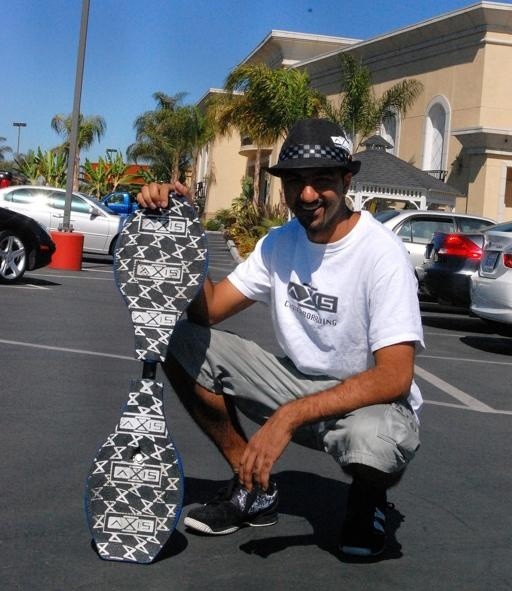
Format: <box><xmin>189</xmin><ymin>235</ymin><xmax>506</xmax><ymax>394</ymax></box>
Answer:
<box><xmin>136</xmin><ymin>120</ymin><xmax>425</xmax><ymax>561</ymax></box>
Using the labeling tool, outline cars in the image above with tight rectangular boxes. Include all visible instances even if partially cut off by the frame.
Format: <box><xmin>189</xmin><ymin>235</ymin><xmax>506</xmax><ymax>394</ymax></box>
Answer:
<box><xmin>0</xmin><ymin>184</ymin><xmax>144</xmax><ymax>289</ymax></box>
<box><xmin>371</xmin><ymin>209</ymin><xmax>512</xmax><ymax>324</ymax></box>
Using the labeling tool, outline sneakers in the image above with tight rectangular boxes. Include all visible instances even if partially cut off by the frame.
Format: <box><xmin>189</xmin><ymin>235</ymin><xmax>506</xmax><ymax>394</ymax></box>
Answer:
<box><xmin>338</xmin><ymin>477</ymin><xmax>387</xmax><ymax>556</ymax></box>
<box><xmin>183</xmin><ymin>479</ymin><xmax>280</xmax><ymax>535</ymax></box>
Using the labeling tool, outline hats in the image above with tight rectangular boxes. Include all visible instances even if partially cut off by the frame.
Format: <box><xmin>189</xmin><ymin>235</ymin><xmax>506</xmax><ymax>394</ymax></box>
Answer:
<box><xmin>267</xmin><ymin>119</ymin><xmax>361</xmax><ymax>176</ymax></box>
